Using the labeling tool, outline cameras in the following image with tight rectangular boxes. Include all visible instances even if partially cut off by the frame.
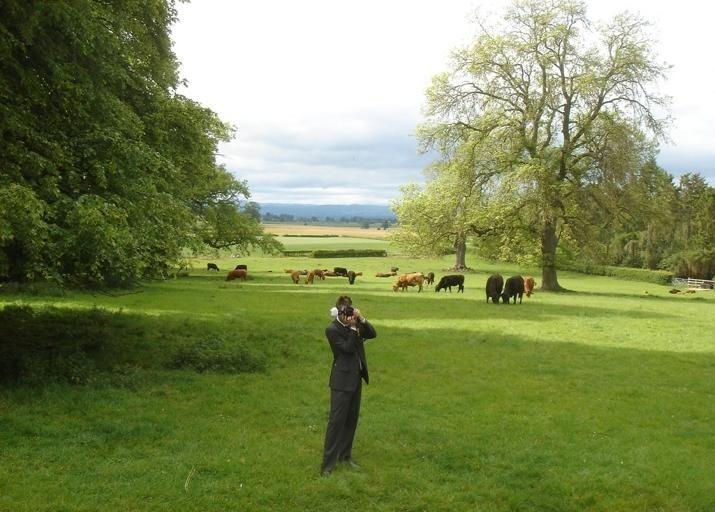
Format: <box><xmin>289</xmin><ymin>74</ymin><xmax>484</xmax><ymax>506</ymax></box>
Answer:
<box><xmin>344</xmin><ymin>307</ymin><xmax>353</xmax><ymax>317</ymax></box>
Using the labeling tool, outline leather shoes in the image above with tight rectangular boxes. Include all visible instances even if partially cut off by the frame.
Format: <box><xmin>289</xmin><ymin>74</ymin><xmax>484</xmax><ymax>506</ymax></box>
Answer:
<box><xmin>321</xmin><ymin>471</ymin><xmax>331</xmax><ymax>478</ymax></box>
<box><xmin>338</xmin><ymin>459</ymin><xmax>360</xmax><ymax>469</ymax></box>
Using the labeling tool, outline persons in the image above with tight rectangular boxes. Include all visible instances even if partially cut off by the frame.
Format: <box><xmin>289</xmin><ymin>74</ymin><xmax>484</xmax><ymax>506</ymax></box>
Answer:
<box><xmin>321</xmin><ymin>296</ymin><xmax>376</xmax><ymax>477</ymax></box>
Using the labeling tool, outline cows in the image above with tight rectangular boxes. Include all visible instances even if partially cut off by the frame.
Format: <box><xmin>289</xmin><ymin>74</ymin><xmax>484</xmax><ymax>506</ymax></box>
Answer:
<box><xmin>207</xmin><ymin>263</ymin><xmax>219</xmax><ymax>271</ymax></box>
<box><xmin>284</xmin><ymin>267</ymin><xmax>363</xmax><ymax>285</ymax></box>
<box><xmin>485</xmin><ymin>273</ymin><xmax>524</xmax><ymax>304</ymax></box>
<box><xmin>374</xmin><ymin>267</ymin><xmax>399</xmax><ymax>278</ymax></box>
<box><xmin>235</xmin><ymin>265</ymin><xmax>247</xmax><ymax>271</ymax></box>
<box><xmin>231</xmin><ymin>252</ymin><xmax>241</xmax><ymax>258</ymax></box>
<box><xmin>224</xmin><ymin>269</ymin><xmax>247</xmax><ymax>282</ymax></box>
<box><xmin>435</xmin><ymin>275</ymin><xmax>465</xmax><ymax>293</ymax></box>
<box><xmin>428</xmin><ymin>273</ymin><xmax>434</xmax><ymax>285</ymax></box>
<box><xmin>393</xmin><ymin>273</ymin><xmax>424</xmax><ymax>293</ymax></box>
<box><xmin>524</xmin><ymin>277</ymin><xmax>537</xmax><ymax>297</ymax></box>
